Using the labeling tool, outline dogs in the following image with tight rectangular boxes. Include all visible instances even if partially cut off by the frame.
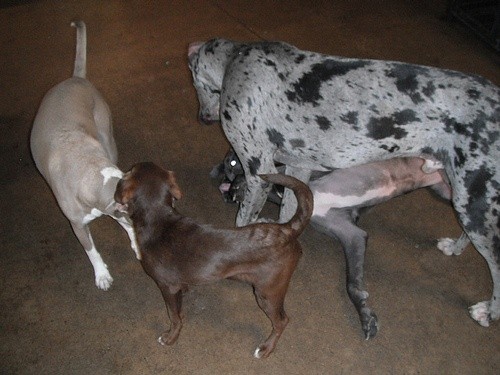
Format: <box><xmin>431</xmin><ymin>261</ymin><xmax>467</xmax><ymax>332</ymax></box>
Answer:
<box><xmin>186</xmin><ymin>36</ymin><xmax>500</xmax><ymax>341</ymax></box>
<box><xmin>115</xmin><ymin>160</ymin><xmax>314</xmax><ymax>360</ymax></box>
<box><xmin>30</xmin><ymin>19</ymin><xmax>144</xmax><ymax>292</ymax></box>
